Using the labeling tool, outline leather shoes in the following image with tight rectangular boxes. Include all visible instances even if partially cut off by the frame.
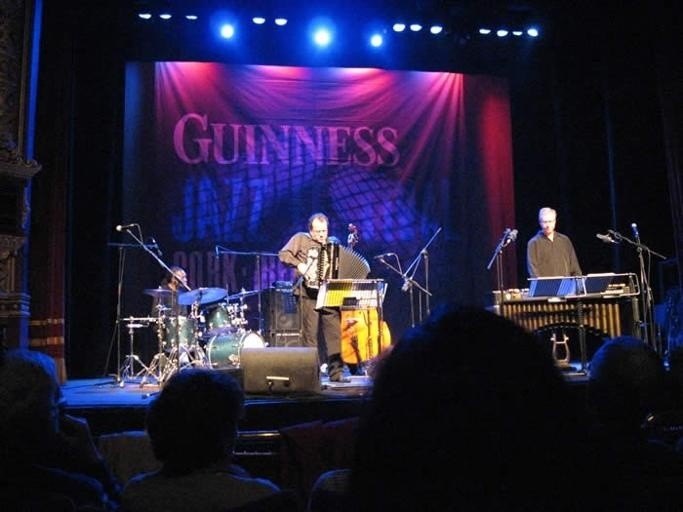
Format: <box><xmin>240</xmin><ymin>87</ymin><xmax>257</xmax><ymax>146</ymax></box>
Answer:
<box><xmin>338</xmin><ymin>377</ymin><xmax>351</xmax><ymax>382</ymax></box>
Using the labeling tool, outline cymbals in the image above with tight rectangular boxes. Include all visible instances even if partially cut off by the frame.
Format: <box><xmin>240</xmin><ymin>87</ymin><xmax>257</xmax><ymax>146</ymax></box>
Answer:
<box><xmin>144</xmin><ymin>289</ymin><xmax>175</xmax><ymax>297</ymax></box>
<box><xmin>177</xmin><ymin>286</ymin><xmax>228</xmax><ymax>305</ymax></box>
<box><xmin>223</xmin><ymin>290</ymin><xmax>258</xmax><ymax>300</ymax></box>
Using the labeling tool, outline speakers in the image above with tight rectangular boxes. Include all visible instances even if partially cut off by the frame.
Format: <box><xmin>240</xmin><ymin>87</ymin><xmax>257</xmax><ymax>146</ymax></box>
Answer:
<box><xmin>240</xmin><ymin>346</ymin><xmax>321</xmax><ymax>397</ymax></box>
<box><xmin>267</xmin><ymin>290</ymin><xmax>302</xmax><ymax>347</ymax></box>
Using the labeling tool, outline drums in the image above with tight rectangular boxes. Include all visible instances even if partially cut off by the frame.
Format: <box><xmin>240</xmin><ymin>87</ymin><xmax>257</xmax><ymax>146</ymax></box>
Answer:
<box><xmin>162</xmin><ymin>315</ymin><xmax>199</xmax><ymax>353</ymax></box>
<box><xmin>203</xmin><ymin>327</ymin><xmax>269</xmax><ymax>371</ymax></box>
<box><xmin>197</xmin><ymin>302</ymin><xmax>240</xmax><ymax>342</ymax></box>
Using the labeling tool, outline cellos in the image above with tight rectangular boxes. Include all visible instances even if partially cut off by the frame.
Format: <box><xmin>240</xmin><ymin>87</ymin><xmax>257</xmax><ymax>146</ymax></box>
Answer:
<box><xmin>338</xmin><ymin>223</ymin><xmax>392</xmax><ymax>365</ymax></box>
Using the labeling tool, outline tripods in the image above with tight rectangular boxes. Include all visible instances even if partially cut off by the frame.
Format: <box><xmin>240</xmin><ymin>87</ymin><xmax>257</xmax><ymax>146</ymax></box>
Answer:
<box><xmin>136</xmin><ymin>299</ymin><xmax>178</xmax><ymax>393</ymax></box>
<box><xmin>161</xmin><ymin>340</ymin><xmax>195</xmax><ymax>386</ymax></box>
<box><xmin>180</xmin><ymin>324</ymin><xmax>212</xmax><ymax>369</ymax></box>
<box><xmin>117</xmin><ymin>330</ymin><xmax>158</xmax><ymax>393</ymax></box>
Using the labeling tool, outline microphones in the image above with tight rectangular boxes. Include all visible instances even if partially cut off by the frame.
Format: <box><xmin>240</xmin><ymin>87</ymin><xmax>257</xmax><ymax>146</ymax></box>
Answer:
<box><xmin>215</xmin><ymin>246</ymin><xmax>219</xmax><ymax>261</ymax></box>
<box><xmin>596</xmin><ymin>233</ymin><xmax>614</xmax><ymax>243</ymax></box>
<box><xmin>402</xmin><ymin>277</ymin><xmax>412</xmax><ymax>292</ymax></box>
<box><xmin>115</xmin><ymin>223</ymin><xmax>134</xmax><ymax>232</ymax></box>
<box><xmin>631</xmin><ymin>223</ymin><xmax>639</xmax><ymax>238</ymax></box>
<box><xmin>151</xmin><ymin>238</ymin><xmax>162</xmax><ymax>257</ymax></box>
<box><xmin>506</xmin><ymin>229</ymin><xmax>518</xmax><ymax>244</ymax></box>
<box><xmin>372</xmin><ymin>253</ymin><xmax>390</xmax><ymax>260</ymax></box>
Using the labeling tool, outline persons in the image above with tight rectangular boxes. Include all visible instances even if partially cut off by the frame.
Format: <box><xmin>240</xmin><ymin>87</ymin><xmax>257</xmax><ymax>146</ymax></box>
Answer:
<box><xmin>527</xmin><ymin>207</ymin><xmax>582</xmax><ymax>278</ymax></box>
<box><xmin>278</xmin><ymin>214</ymin><xmax>352</xmax><ymax>383</ymax></box>
<box><xmin>0</xmin><ymin>349</ymin><xmax>285</xmax><ymax>512</ymax></box>
<box><xmin>149</xmin><ymin>266</ymin><xmax>208</xmax><ymax>365</ymax></box>
<box><xmin>337</xmin><ymin>304</ymin><xmax>683</xmax><ymax>512</ymax></box>
